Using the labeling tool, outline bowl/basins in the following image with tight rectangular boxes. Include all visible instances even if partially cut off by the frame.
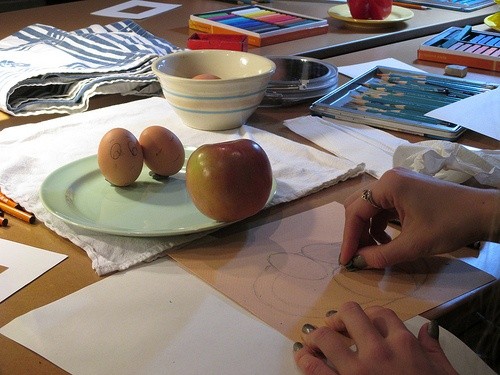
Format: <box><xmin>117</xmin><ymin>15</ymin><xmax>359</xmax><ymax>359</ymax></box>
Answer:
<box><xmin>151</xmin><ymin>50</ymin><xmax>276</xmax><ymax>130</ymax></box>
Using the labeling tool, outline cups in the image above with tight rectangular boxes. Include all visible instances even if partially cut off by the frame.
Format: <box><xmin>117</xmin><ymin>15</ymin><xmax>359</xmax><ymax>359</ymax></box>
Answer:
<box><xmin>347</xmin><ymin>0</ymin><xmax>393</xmax><ymax>20</ymax></box>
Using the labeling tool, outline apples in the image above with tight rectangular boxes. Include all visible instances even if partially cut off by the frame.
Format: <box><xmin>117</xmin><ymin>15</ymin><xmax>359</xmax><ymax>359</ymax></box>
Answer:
<box><xmin>186</xmin><ymin>138</ymin><xmax>272</xmax><ymax>222</ymax></box>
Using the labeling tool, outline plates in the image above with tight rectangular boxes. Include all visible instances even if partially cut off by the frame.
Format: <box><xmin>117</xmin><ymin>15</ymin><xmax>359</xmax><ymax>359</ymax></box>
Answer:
<box><xmin>328</xmin><ymin>4</ymin><xmax>415</xmax><ymax>29</ymax></box>
<box><xmin>483</xmin><ymin>11</ymin><xmax>500</xmax><ymax>31</ymax></box>
<box><xmin>39</xmin><ymin>147</ymin><xmax>277</xmax><ymax>237</ymax></box>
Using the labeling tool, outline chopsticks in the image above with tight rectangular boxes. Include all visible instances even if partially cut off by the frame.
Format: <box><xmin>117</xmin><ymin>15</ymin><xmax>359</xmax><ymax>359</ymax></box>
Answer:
<box><xmin>0</xmin><ymin>193</ymin><xmax>36</xmax><ymax>226</ymax></box>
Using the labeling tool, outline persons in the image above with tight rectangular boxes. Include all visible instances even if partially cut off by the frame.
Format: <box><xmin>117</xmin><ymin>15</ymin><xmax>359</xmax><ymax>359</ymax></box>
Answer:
<box><xmin>293</xmin><ymin>167</ymin><xmax>500</xmax><ymax>375</ymax></box>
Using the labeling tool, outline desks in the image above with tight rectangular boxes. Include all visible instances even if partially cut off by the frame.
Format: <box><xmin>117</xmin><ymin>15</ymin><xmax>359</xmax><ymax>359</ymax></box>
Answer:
<box><xmin>0</xmin><ymin>0</ymin><xmax>499</xmax><ymax>375</ymax></box>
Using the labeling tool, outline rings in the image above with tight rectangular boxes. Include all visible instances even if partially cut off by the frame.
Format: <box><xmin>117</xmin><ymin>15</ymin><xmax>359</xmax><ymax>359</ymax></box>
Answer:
<box><xmin>361</xmin><ymin>188</ymin><xmax>382</xmax><ymax>210</ymax></box>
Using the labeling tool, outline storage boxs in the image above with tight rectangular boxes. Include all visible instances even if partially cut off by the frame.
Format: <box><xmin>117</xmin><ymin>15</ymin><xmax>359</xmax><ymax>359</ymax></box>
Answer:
<box><xmin>188</xmin><ymin>4</ymin><xmax>330</xmax><ymax>47</ymax></box>
<box><xmin>418</xmin><ymin>26</ymin><xmax>500</xmax><ymax>71</ymax></box>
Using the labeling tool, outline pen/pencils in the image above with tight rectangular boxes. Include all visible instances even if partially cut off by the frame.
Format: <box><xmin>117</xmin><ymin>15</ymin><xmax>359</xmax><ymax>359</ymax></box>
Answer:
<box><xmin>0</xmin><ymin>189</ymin><xmax>35</xmax><ymax>227</ymax></box>
<box><xmin>392</xmin><ymin>1</ymin><xmax>431</xmax><ymax>10</ymax></box>
<box><xmin>349</xmin><ymin>74</ymin><xmax>496</xmax><ymax>129</ymax></box>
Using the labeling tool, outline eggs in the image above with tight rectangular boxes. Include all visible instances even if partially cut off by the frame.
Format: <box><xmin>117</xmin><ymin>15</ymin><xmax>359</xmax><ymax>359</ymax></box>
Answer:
<box><xmin>98</xmin><ymin>127</ymin><xmax>143</xmax><ymax>186</ymax></box>
<box><xmin>138</xmin><ymin>126</ymin><xmax>185</xmax><ymax>176</ymax></box>
<box><xmin>190</xmin><ymin>73</ymin><xmax>221</xmax><ymax>79</ymax></box>
